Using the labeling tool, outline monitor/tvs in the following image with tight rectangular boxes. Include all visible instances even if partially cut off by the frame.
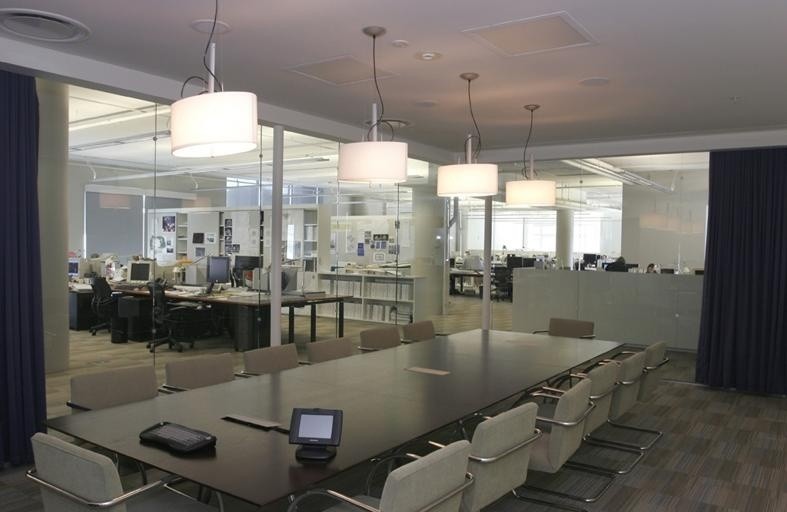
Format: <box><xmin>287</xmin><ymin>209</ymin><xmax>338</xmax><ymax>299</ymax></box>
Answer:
<box><xmin>205</xmin><ymin>256</ymin><xmax>230</xmax><ymax>293</ymax></box>
<box><xmin>127</xmin><ymin>260</ymin><xmax>164</xmax><ymax>287</ymax></box>
<box><xmin>287</xmin><ymin>407</ymin><xmax>343</xmax><ymax>461</ymax></box>
<box><xmin>234</xmin><ymin>255</ymin><xmax>263</xmax><ymax>276</ymax></box>
<box><xmin>89</xmin><ymin>257</ymin><xmax>101</xmax><ymax>276</ymax></box>
<box><xmin>67</xmin><ymin>257</ymin><xmax>83</xmax><ymax>282</ymax></box>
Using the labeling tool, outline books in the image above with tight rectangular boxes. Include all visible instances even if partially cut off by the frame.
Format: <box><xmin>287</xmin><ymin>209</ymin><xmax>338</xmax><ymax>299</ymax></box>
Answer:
<box><xmin>366</xmin><ymin>304</ymin><xmax>411</xmax><ymax>324</ymax></box>
<box><xmin>319</xmin><ymin>280</ymin><xmax>360</xmax><ymax>297</ymax></box>
<box><xmin>316</xmin><ymin>302</ymin><xmax>361</xmax><ymax>319</ymax></box>
<box><xmin>366</xmin><ymin>281</ymin><xmax>412</xmax><ymax>301</ymax></box>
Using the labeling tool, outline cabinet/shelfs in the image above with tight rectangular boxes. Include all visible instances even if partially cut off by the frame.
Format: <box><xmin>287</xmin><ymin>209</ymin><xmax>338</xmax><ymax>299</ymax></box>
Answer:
<box><xmin>148</xmin><ymin>202</ymin><xmax>331</xmax><ymax>293</ymax></box>
<box><xmin>317</xmin><ymin>272</ymin><xmax>415</xmax><ymax>324</ymax></box>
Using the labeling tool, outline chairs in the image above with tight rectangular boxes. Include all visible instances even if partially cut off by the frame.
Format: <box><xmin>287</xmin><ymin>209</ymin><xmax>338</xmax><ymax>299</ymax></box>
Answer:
<box><xmin>26</xmin><ymin>433</ymin><xmax>217</xmax><ymax>511</ymax></box>
<box><xmin>464</xmin><ymin>254</ymin><xmax>535</xmax><ymax>302</ymax></box>
<box><xmin>533</xmin><ymin>317</ymin><xmax>594</xmax><ymax>405</ymax></box>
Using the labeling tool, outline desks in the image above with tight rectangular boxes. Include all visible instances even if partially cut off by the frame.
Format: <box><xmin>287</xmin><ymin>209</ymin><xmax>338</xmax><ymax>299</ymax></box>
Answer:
<box><xmin>68</xmin><ymin>281</ymin><xmax>352</xmax><ymax>353</ymax></box>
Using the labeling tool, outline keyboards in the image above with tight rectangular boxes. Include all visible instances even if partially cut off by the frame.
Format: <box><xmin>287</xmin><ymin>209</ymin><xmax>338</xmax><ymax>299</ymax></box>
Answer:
<box><xmin>138</xmin><ymin>420</ymin><xmax>218</xmax><ymax>456</ymax></box>
<box><xmin>116</xmin><ymin>285</ymin><xmax>143</xmax><ymax>290</ymax></box>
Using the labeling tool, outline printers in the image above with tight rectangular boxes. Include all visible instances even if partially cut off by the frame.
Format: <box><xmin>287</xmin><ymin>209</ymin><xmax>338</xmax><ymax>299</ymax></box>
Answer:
<box><xmin>180</xmin><ymin>262</ymin><xmax>207</xmax><ymax>285</ymax></box>
<box><xmin>253</xmin><ymin>265</ymin><xmax>301</xmax><ymax>294</ymax></box>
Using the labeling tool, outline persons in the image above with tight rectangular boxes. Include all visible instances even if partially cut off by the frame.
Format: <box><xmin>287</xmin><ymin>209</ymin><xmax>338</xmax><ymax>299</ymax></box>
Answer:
<box><xmin>647</xmin><ymin>264</ymin><xmax>657</xmax><ymax>273</ymax></box>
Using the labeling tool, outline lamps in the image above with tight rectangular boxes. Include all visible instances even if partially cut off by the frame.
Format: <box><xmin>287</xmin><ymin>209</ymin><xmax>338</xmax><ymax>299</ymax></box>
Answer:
<box><xmin>170</xmin><ymin>1</ymin><xmax>258</xmax><ymax>158</ymax></box>
<box><xmin>438</xmin><ymin>72</ymin><xmax>499</xmax><ymax>198</ymax></box>
<box><xmin>506</xmin><ymin>104</ymin><xmax>555</xmax><ymax>207</ymax></box>
<box><xmin>337</xmin><ymin>26</ymin><xmax>407</xmax><ymax>184</ymax></box>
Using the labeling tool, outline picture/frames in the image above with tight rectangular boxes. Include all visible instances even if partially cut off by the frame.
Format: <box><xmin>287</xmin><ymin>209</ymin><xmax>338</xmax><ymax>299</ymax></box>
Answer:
<box><xmin>373</xmin><ymin>234</ymin><xmax>389</xmax><ymax>263</ymax></box>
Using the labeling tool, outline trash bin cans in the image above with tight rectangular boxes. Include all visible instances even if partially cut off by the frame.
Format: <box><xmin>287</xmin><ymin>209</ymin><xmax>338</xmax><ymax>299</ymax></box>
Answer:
<box><xmin>111</xmin><ymin>316</ymin><xmax>128</xmax><ymax>344</ymax></box>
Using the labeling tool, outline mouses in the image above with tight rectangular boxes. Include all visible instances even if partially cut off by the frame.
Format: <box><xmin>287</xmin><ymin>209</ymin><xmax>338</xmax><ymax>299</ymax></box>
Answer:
<box><xmin>134</xmin><ymin>288</ymin><xmax>140</xmax><ymax>291</ymax></box>
